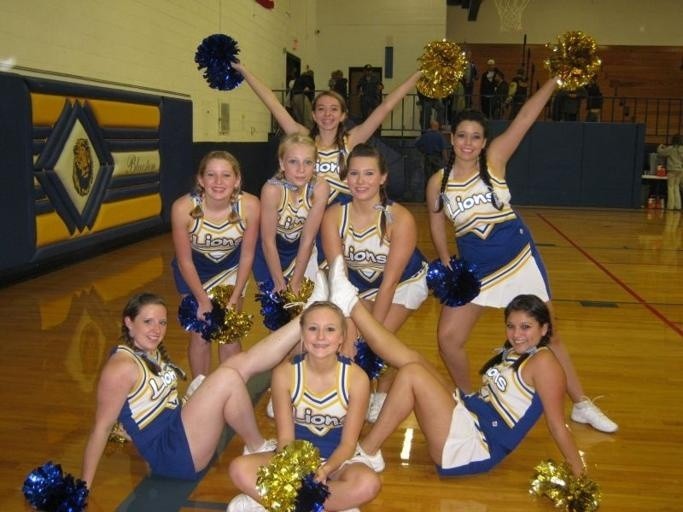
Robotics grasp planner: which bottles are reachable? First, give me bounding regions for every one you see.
[657,163,666,176]
[648,194,665,209]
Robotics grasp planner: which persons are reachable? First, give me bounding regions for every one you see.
[656,134,682,210]
[170,151,263,383]
[419,120,448,177]
[81,269,329,490]
[417,59,603,129]
[425,73,618,433]
[288,63,384,135]
[330,254,586,481]
[320,144,430,424]
[661,210,682,263]
[232,61,426,204]
[251,132,330,419]
[226,300,381,512]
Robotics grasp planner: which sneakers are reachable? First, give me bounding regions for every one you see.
[366,392,388,423]
[306,253,359,317]
[354,442,385,472]
[571,398,618,433]
[267,396,274,418]
[242,438,278,455]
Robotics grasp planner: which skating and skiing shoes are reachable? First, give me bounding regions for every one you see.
[226,493,273,512]
